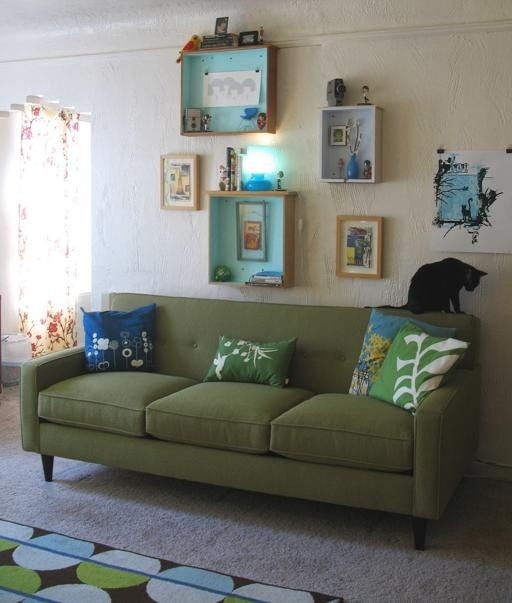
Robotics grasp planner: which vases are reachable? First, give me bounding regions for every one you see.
[347,155,359,179]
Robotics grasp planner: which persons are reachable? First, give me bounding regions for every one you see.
[217,21,226,33]
[245,226,258,249]
[206,77,257,105]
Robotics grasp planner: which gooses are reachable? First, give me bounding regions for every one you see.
[462,198,473,221]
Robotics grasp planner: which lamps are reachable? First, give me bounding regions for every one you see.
[241,145,276,191]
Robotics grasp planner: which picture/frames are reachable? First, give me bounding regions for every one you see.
[238,30,258,46]
[159,154,200,211]
[234,200,267,262]
[336,215,383,280]
[329,125,347,146]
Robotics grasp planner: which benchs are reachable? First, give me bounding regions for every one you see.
[19,290,483,549]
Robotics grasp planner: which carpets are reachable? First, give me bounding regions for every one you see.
[0,518,343,603]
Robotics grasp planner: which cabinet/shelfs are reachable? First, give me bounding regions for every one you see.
[204,190,298,289]
[318,105,384,184]
[179,43,277,137]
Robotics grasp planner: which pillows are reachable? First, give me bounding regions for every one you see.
[348,306,457,396]
[80,302,157,373]
[368,317,473,417]
[202,334,299,389]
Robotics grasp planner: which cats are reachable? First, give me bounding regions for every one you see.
[364,257,488,314]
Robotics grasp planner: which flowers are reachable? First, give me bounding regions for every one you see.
[344,117,362,154]
